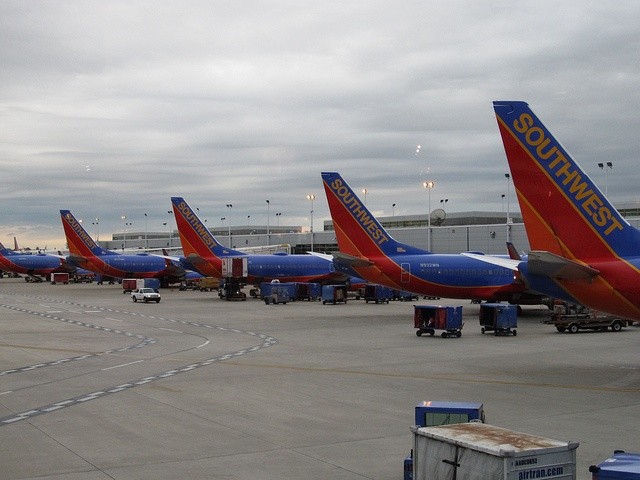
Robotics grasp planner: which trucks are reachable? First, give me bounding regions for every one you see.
[264,288,289,304]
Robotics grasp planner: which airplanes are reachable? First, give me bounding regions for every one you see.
[173,197,383,287]
[320,172,528,304]
[493,101,640,321]
[60,210,203,279]
[0,237,93,277]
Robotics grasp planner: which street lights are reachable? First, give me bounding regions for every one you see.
[392,203,396,215]
[501,194,505,212]
[307,194,315,251]
[226,204,232,249]
[220,218,226,227]
[163,223,167,232]
[361,188,368,207]
[504,172,510,255]
[266,199,270,246]
[144,214,147,249]
[422,182,434,252]
[126,223,132,239]
[441,198,449,214]
[204,220,207,225]
[276,212,281,227]
[92,222,99,242]
[196,208,200,217]
[96,217,100,242]
[598,162,613,198]
[168,210,173,247]
[248,215,250,235]
[121,216,126,249]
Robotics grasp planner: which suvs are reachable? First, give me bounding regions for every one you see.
[551,297,626,333]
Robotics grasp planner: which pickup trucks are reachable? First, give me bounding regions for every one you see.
[131,288,161,303]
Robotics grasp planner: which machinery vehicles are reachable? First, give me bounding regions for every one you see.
[145,279,160,293]
[295,283,319,301]
[122,279,145,294]
[51,273,69,285]
[413,304,465,339]
[322,285,348,305]
[589,449,640,480]
[480,304,517,336]
[404,401,579,480]
[364,284,390,304]
[199,277,220,292]
[260,282,296,302]
[389,288,419,302]
[218,276,246,301]
